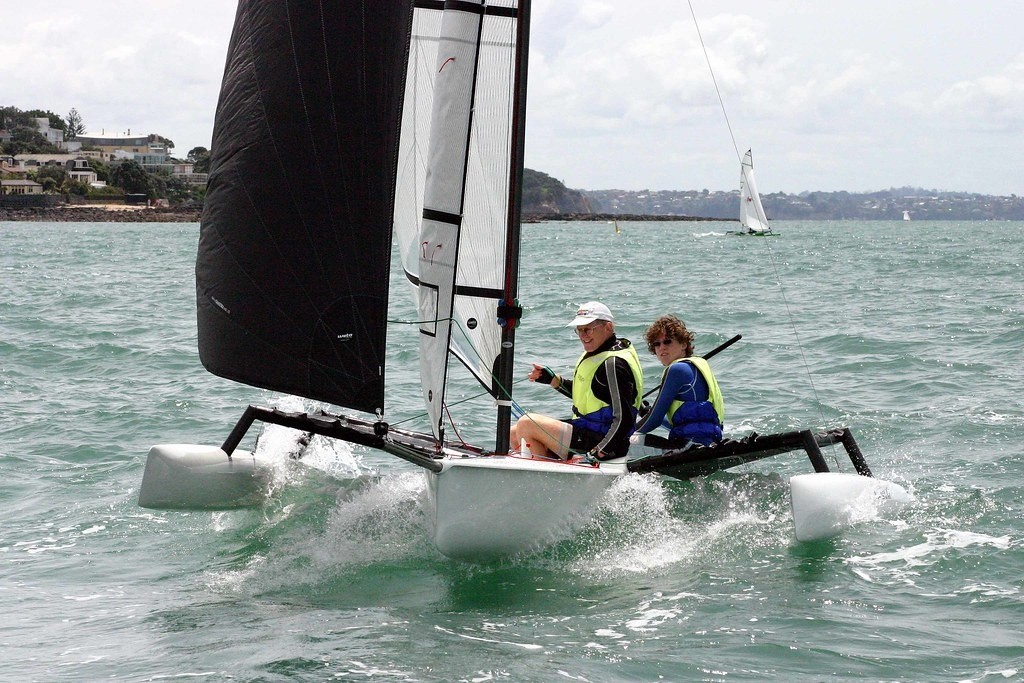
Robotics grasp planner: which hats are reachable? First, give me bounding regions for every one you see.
[566,301,614,328]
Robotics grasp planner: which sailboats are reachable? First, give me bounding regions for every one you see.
[722,145,783,240]
[130,1,922,563]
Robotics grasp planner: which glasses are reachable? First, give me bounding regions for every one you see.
[653,339,673,347]
[574,323,603,336]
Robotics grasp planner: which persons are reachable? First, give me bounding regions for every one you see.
[509,301,644,464]
[630,313,725,459]
